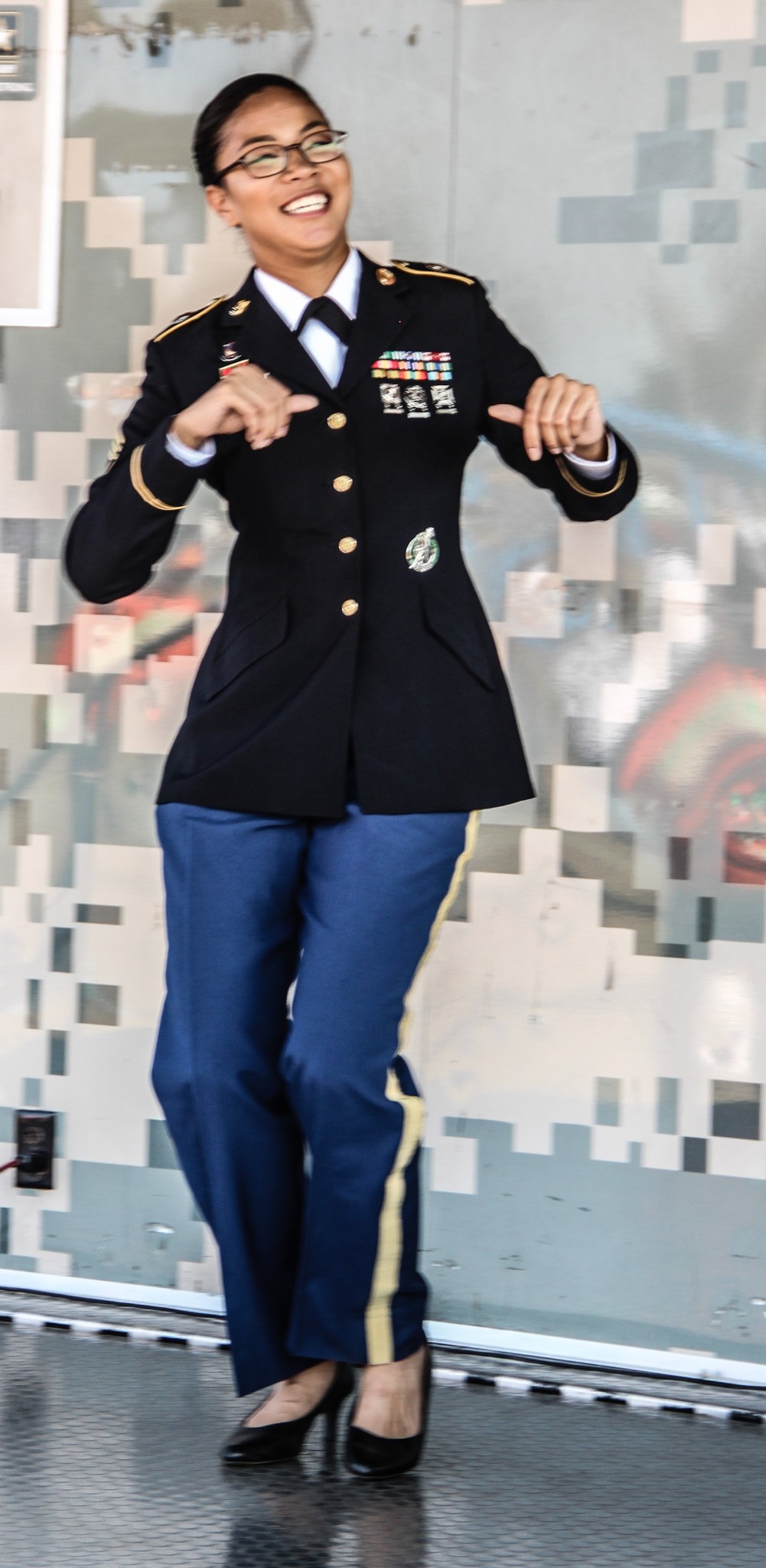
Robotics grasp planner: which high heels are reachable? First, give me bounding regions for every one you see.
[343,1342,431,1478]
[218,1361,355,1465]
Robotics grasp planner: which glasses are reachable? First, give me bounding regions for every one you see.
[201,129,348,185]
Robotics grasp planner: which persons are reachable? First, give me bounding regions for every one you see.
[66,74,640,1492]
